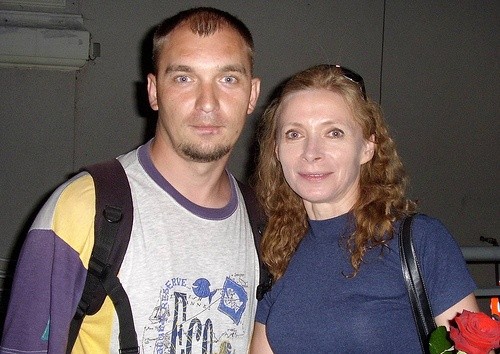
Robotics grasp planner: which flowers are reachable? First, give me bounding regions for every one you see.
[426,309,500,354]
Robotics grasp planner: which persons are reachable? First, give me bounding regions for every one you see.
[1,7,272,353]
[250,64,493,354]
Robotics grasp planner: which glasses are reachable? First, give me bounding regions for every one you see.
[321,64,368,104]
